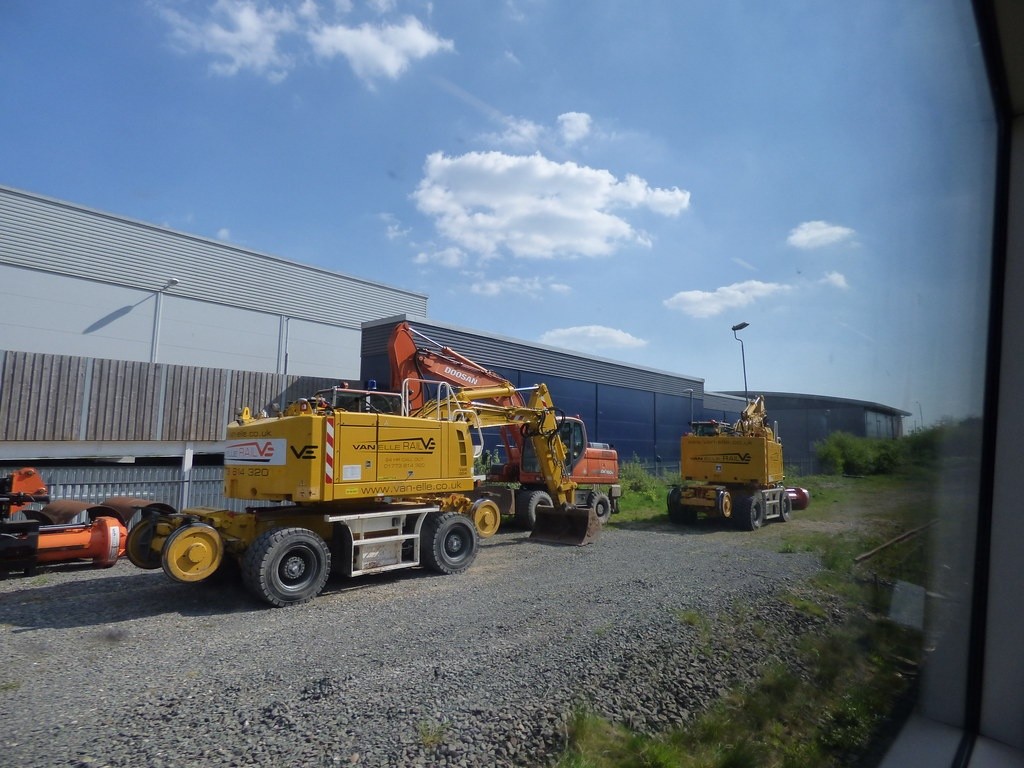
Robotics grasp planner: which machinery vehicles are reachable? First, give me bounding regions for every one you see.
[665,394,793,531]
[129,379,601,608]
[375,322,622,528]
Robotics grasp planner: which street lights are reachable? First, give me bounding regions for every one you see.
[732,321,751,410]
